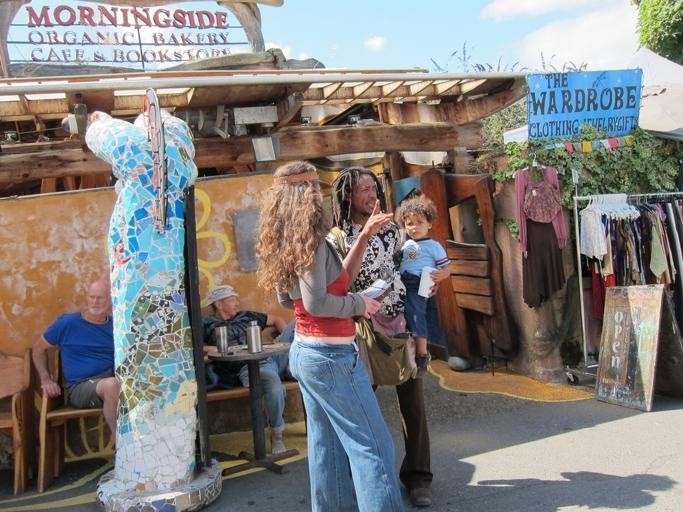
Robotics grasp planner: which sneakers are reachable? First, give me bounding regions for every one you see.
[414,351,432,380]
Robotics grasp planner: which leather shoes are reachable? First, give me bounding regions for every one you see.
[411,488,432,506]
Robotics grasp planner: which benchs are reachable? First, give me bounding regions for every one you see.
[36,341,300,493]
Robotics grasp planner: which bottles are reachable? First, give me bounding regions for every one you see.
[245,318,262,354]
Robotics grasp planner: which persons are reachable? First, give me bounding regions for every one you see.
[30,279,119,440]
[199,284,296,455]
[391,196,452,379]
[322,165,435,508]
[254,160,407,511]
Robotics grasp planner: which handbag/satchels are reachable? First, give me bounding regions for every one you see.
[356,313,419,386]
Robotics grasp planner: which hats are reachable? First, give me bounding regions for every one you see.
[200,285,238,308]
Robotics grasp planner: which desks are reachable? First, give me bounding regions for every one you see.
[205,345,304,477]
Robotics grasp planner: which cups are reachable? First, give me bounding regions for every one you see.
[417,266,437,299]
[214,326,229,354]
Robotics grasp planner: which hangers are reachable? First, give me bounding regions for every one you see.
[575,189,682,221]
[506,152,564,179]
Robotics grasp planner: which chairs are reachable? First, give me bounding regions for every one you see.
[0,348,31,496]
